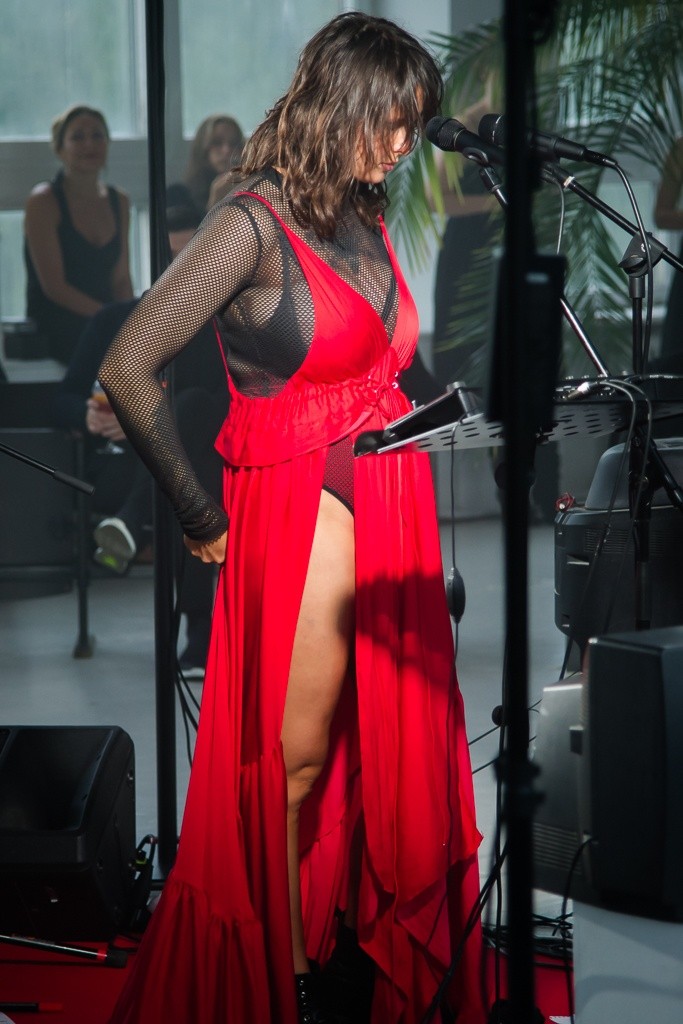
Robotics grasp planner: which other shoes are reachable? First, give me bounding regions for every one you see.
[93,517,137,574]
[293,974,316,1024]
[178,652,206,677]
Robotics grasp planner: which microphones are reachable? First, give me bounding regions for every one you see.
[479,114,617,167]
[426,115,556,183]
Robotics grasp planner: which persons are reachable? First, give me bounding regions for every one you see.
[23,107,248,678]
[98,12,489,1024]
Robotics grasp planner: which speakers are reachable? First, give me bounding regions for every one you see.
[0,724,137,942]
[584,625,683,926]
